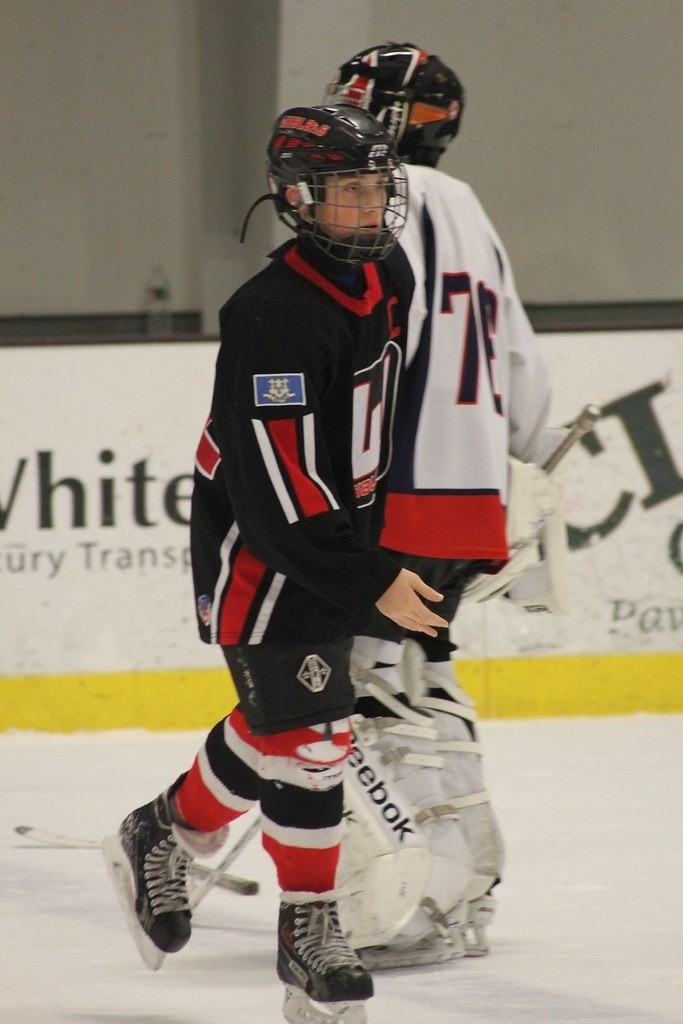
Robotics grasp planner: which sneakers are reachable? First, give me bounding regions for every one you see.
[347,897,493,970]
[103,770,231,971]
[277,886,373,1024]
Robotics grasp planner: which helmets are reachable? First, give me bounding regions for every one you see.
[323,42,465,168]
[268,103,410,264]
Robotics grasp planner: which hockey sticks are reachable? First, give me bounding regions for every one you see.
[10,404,599,970]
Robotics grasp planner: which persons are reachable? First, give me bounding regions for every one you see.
[103,100,447,1024]
[325,44,570,974]
